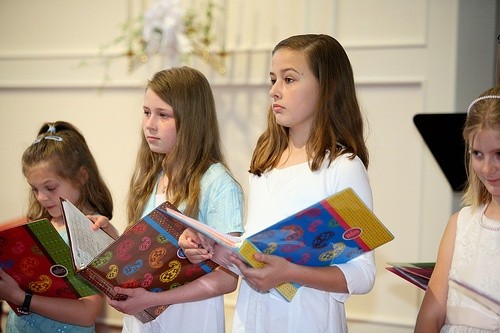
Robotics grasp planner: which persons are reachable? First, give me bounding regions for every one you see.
[86,65,244,333]
[412,87,500,333]
[177,33,376,333]
[0,121,104,333]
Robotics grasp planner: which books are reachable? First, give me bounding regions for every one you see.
[448,279,500,316]
[58,196,220,324]
[384,260,437,292]
[157,186,395,303]
[0,218,102,300]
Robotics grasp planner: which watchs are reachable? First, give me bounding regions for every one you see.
[16,290,33,314]
